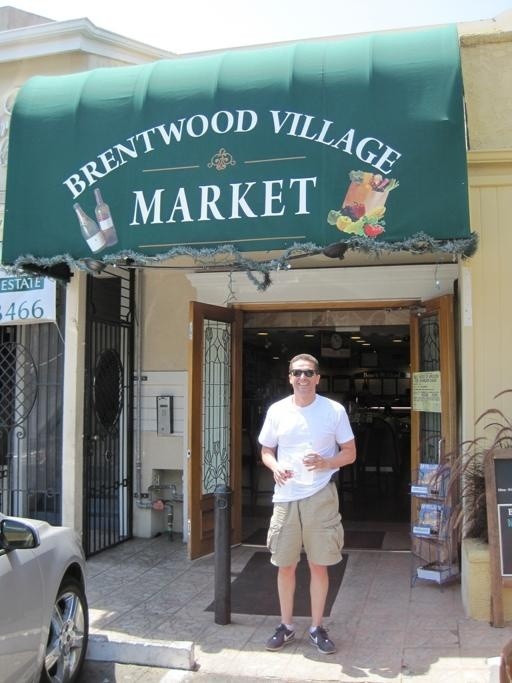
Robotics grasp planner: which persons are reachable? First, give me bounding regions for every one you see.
[257,353,358,655]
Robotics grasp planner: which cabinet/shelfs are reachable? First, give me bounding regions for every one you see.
[408,433,460,594]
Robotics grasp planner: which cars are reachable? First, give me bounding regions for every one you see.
[0,508,89,683]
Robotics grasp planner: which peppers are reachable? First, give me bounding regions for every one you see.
[327,202,383,237]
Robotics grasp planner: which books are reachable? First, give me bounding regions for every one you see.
[410,462,459,583]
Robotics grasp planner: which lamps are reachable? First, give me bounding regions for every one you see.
[85,258,130,281]
[322,242,347,260]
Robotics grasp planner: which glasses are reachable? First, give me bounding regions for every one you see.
[288,369,314,378]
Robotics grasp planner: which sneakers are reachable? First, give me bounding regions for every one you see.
[264,624,296,651]
[308,625,337,654]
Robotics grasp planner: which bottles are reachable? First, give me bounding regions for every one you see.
[302,442,313,481]
[72,202,106,255]
[93,188,119,248]
